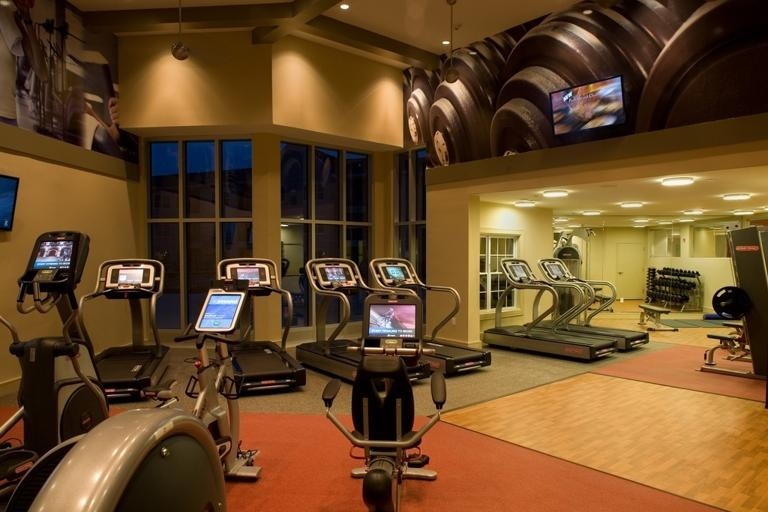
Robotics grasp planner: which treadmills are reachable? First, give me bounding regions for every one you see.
[296,257,492,385]
[481,257,649,361]
[215,257,307,393]
[77,258,172,403]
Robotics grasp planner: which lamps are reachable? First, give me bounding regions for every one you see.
[445,0,460,84]
[171,0,189,60]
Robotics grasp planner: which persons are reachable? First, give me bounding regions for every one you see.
[0,0,121,153]
[380,305,400,322]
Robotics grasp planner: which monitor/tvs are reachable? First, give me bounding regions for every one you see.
[376,263,415,284]
[105,263,155,291]
[363,299,424,341]
[547,74,636,148]
[225,263,271,285]
[195,288,245,332]
[542,262,568,279]
[315,263,358,287]
[0,174,20,231]
[30,238,76,274]
[506,262,533,282]
